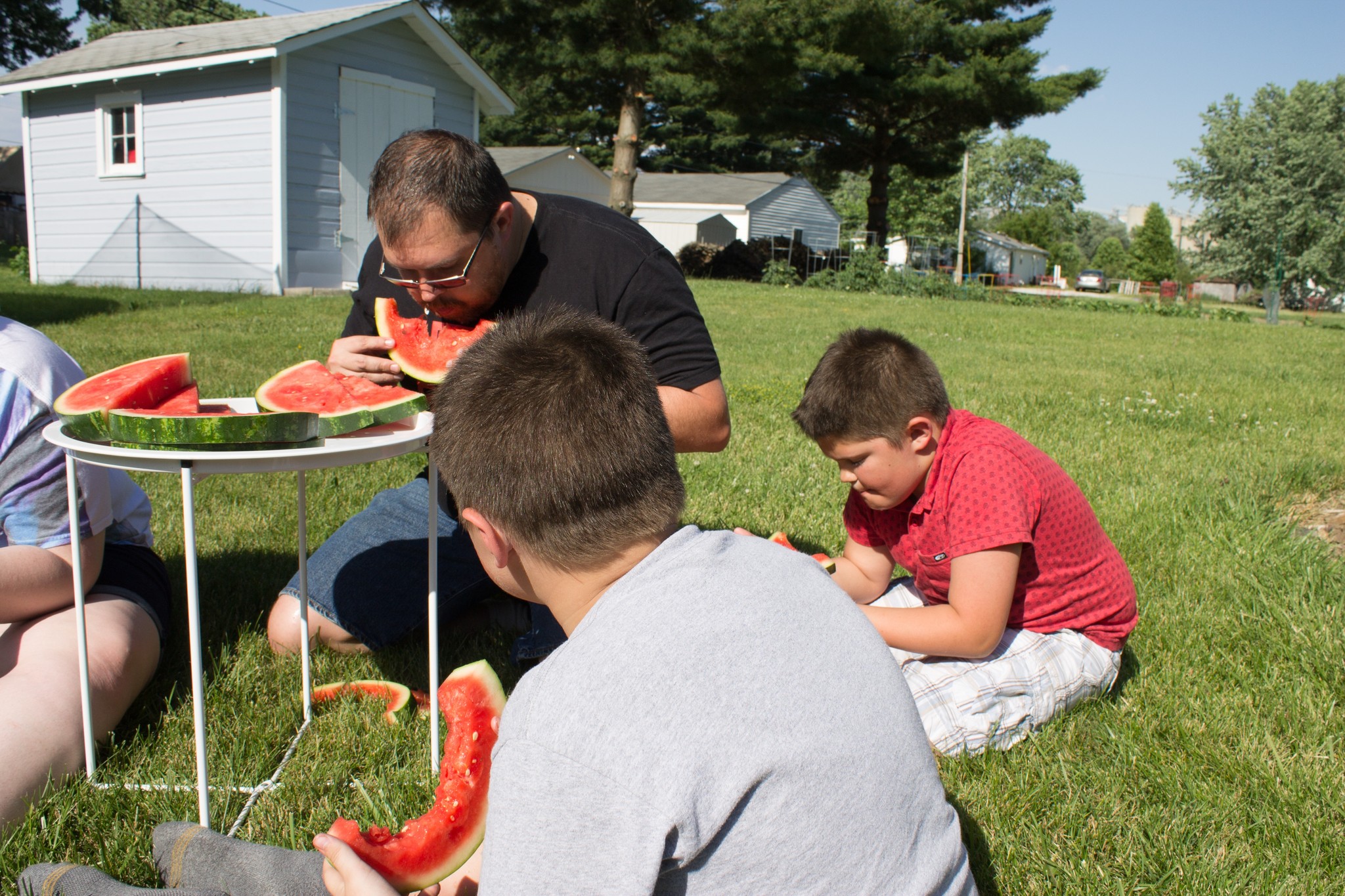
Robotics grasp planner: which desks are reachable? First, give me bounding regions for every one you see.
[40,394,440,839]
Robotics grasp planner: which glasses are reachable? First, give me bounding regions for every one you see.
[379,218,492,289]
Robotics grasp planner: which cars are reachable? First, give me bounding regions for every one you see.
[1076,269,1111,294]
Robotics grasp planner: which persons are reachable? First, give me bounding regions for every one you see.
[18,308,981,896]
[733,326,1141,757]
[268,132,731,694]
[0,315,169,838]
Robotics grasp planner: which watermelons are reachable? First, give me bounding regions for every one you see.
[52,352,325,451]
[331,370,430,430]
[374,297,500,385]
[328,657,508,893]
[767,531,836,575]
[254,358,374,438]
